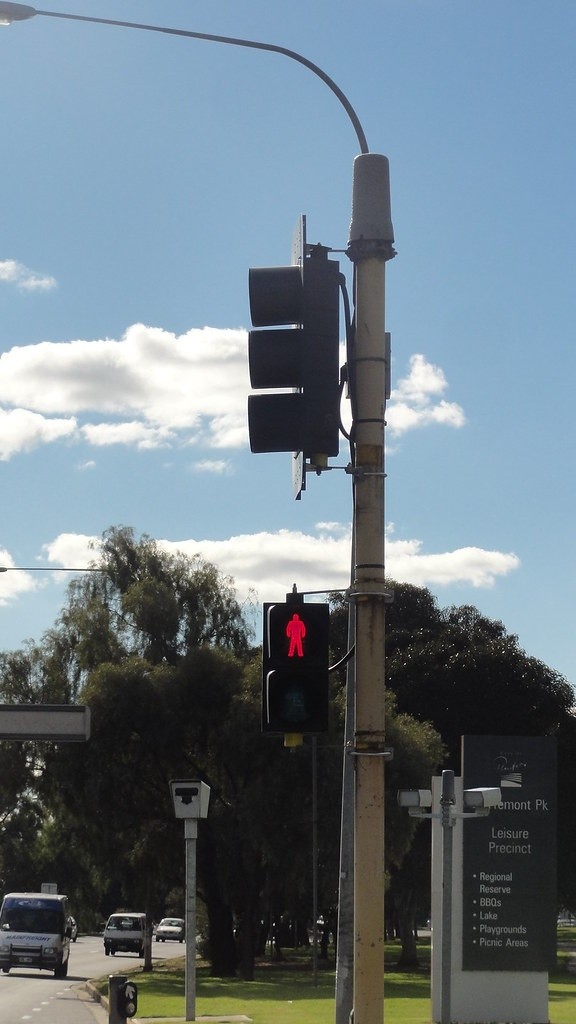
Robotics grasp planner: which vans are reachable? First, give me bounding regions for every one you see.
[103,911,146,958]
[0,892,69,978]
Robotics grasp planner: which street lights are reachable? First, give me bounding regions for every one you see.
[0,3,383,1024]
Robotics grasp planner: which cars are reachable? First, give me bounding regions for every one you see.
[70,916,78,943]
[156,918,184,943]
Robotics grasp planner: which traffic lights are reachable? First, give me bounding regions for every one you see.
[250,214,337,498]
[262,603,334,736]
